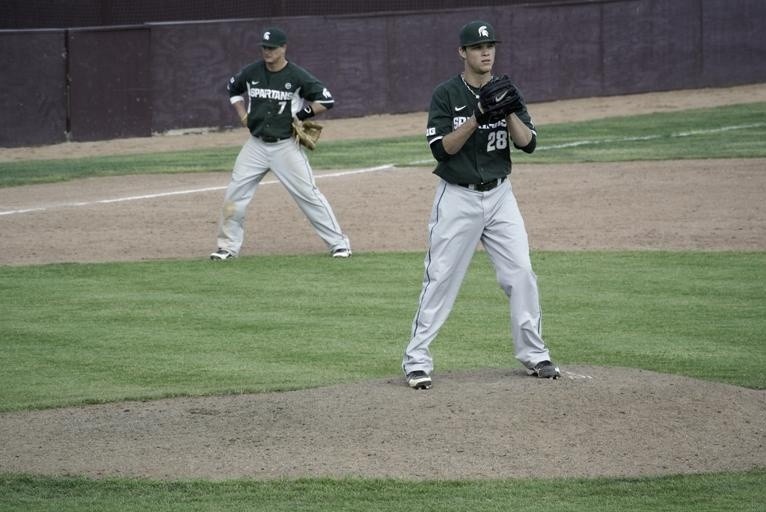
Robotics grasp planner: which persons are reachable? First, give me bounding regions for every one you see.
[399,21,567,392]
[207,28,354,261]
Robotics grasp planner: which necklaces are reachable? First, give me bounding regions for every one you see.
[458,72,495,98]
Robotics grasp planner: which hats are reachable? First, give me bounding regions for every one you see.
[254,29,287,48]
[459,20,502,46]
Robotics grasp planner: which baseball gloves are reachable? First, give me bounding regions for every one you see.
[475,74,521,125]
[294,119,323,150]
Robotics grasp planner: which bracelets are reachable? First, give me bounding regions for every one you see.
[241,112,248,123]
[296,105,316,122]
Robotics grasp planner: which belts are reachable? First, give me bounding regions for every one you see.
[253,134,289,142]
[457,176,505,191]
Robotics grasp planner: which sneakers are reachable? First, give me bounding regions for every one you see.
[209,250,232,260]
[404,370,431,390]
[526,360,560,378]
[332,248,351,258]
